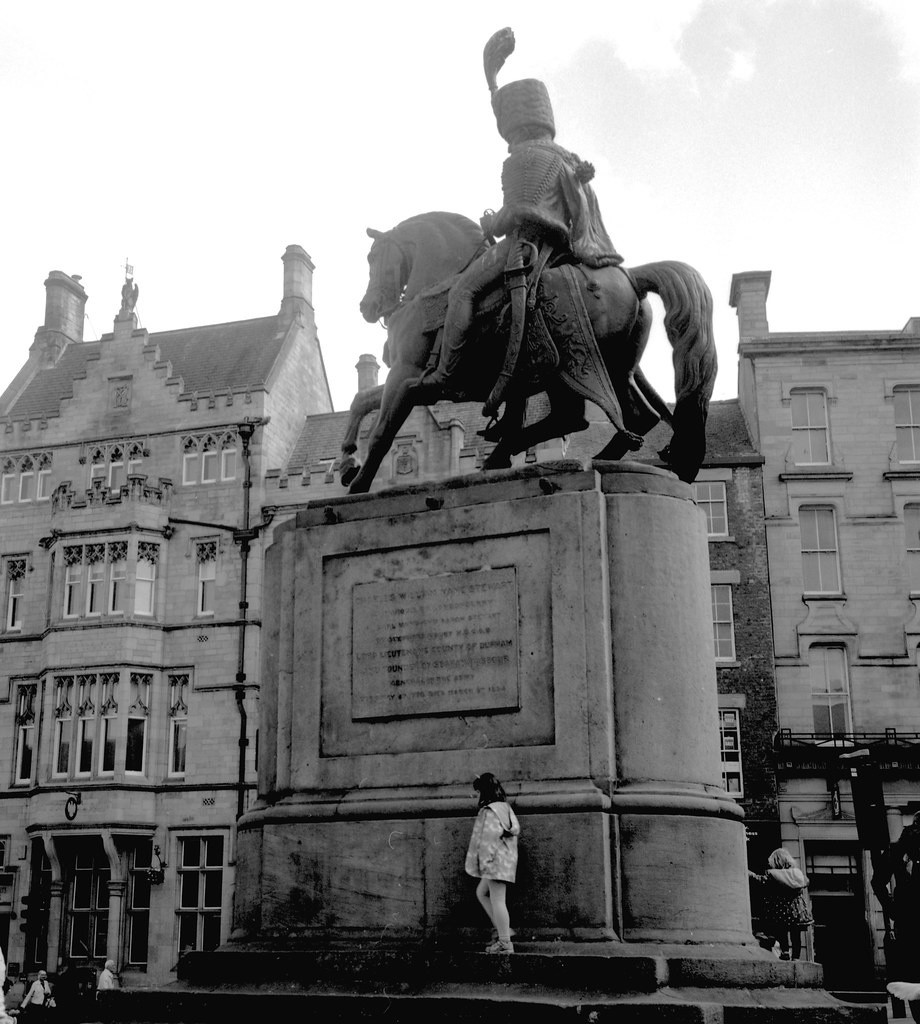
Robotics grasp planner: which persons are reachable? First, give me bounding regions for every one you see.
[763,847,815,961]
[406,125,625,389]
[19,970,51,1024]
[463,774,521,956]
[95,959,116,1000]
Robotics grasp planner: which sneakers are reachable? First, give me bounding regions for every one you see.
[484,937,515,955]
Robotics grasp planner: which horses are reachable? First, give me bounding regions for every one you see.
[337,209,719,486]
